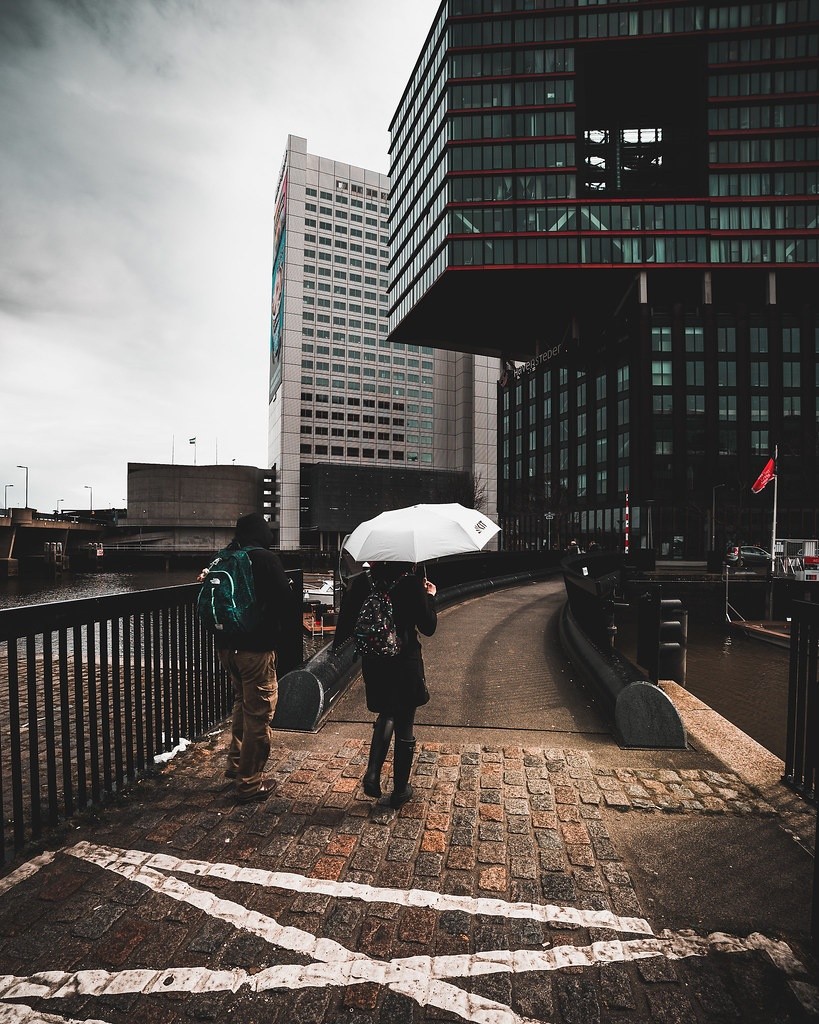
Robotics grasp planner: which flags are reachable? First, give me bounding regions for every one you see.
[751,458,775,493]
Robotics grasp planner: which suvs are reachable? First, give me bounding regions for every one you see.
[725,546,776,567]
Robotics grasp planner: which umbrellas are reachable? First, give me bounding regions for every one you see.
[344,502,502,577]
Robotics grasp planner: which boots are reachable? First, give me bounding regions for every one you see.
[389,738,418,805]
[363,736,390,798]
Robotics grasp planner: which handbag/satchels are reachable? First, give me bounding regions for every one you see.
[577,545,581,554]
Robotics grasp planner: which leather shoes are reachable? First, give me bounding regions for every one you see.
[239,778,278,804]
[225,770,238,779]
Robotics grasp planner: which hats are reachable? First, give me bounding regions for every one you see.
[571,541,577,544]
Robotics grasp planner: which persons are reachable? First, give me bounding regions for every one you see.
[567,541,580,554]
[727,543,738,574]
[340,562,437,807]
[590,541,602,551]
[217,514,297,802]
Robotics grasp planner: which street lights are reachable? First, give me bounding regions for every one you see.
[5,485,13,517]
[712,484,724,550]
[17,466,27,508]
[86,486,92,510]
[544,512,555,550]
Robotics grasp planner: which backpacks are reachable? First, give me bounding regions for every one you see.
[193,543,266,638]
[350,570,413,663]
